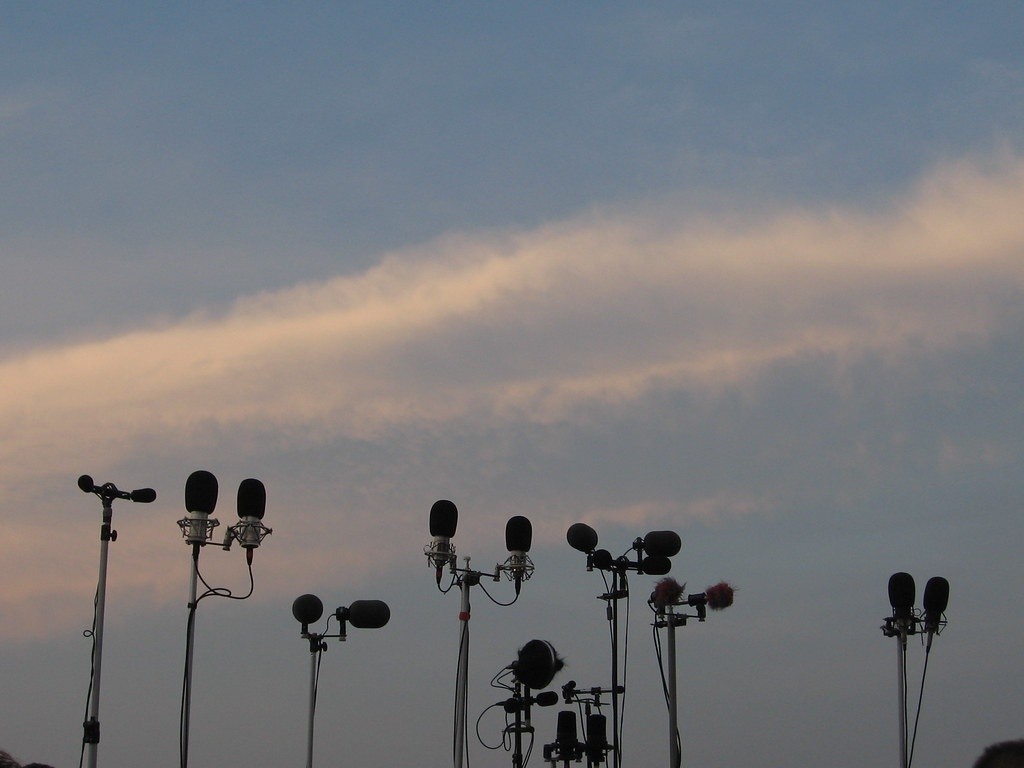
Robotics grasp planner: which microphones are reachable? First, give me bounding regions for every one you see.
[131,488,156,503]
[923,577,950,651]
[567,523,733,609]
[292,594,325,625]
[336,600,390,628]
[237,479,266,563]
[498,640,607,768]
[78,475,94,492]
[430,500,458,584]
[506,516,532,594]
[185,470,219,560]
[889,572,916,648]
